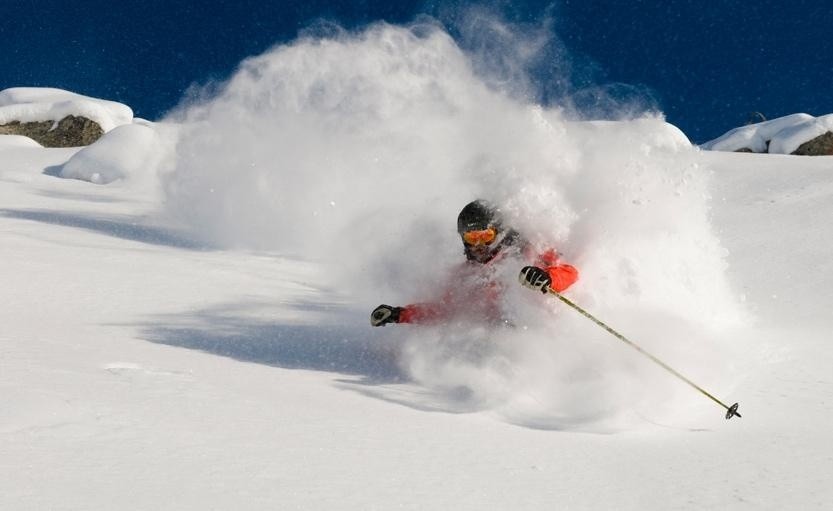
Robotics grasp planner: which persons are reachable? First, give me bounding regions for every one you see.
[368,197,580,333]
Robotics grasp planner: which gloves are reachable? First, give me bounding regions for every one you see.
[370,304,400,327]
[518,266,552,294]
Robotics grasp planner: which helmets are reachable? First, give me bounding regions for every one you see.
[456,199,502,236]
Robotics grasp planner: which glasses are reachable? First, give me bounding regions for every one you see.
[459,227,500,245]
